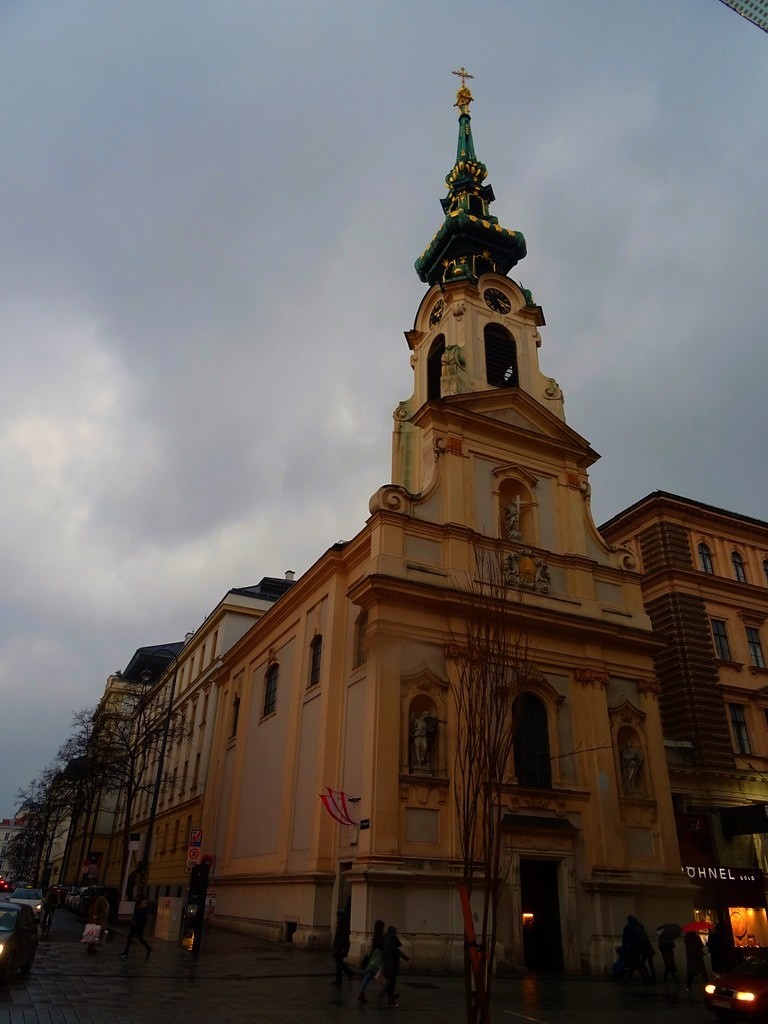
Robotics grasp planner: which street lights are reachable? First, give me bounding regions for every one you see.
[136,649,179,907]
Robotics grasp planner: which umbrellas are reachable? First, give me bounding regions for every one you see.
[655,923,683,940]
[681,921,714,933]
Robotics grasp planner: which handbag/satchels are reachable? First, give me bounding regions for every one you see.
[80,918,102,945]
[369,949,384,973]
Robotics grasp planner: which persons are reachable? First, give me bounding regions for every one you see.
[504,498,519,533]
[658,936,683,986]
[86,888,110,954]
[685,931,710,992]
[412,711,430,765]
[706,923,730,976]
[330,909,353,987]
[613,914,656,986]
[621,740,644,791]
[357,919,413,1007]
[117,890,153,960]
[40,886,57,928]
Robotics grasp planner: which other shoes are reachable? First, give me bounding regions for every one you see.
[331,980,342,987]
[119,953,128,957]
[384,999,400,1008]
[347,970,354,982]
[358,993,368,1004]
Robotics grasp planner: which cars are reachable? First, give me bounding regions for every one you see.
[0,902,39,987]
[6,885,46,924]
[703,949,768,1024]
[54,883,120,922]
